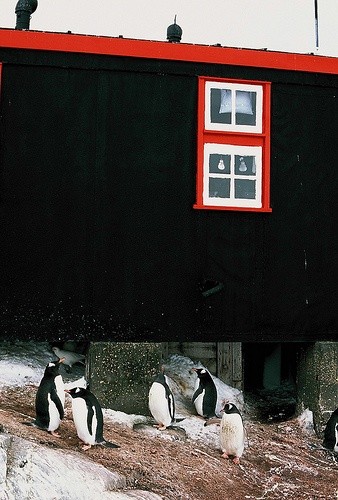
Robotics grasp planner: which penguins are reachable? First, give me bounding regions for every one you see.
[322,408,338,453]
[64,387,121,451]
[204,403,249,464]
[21,357,65,438]
[148,367,187,431]
[190,368,218,421]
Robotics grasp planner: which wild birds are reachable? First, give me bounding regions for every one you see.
[50,346,86,374]
